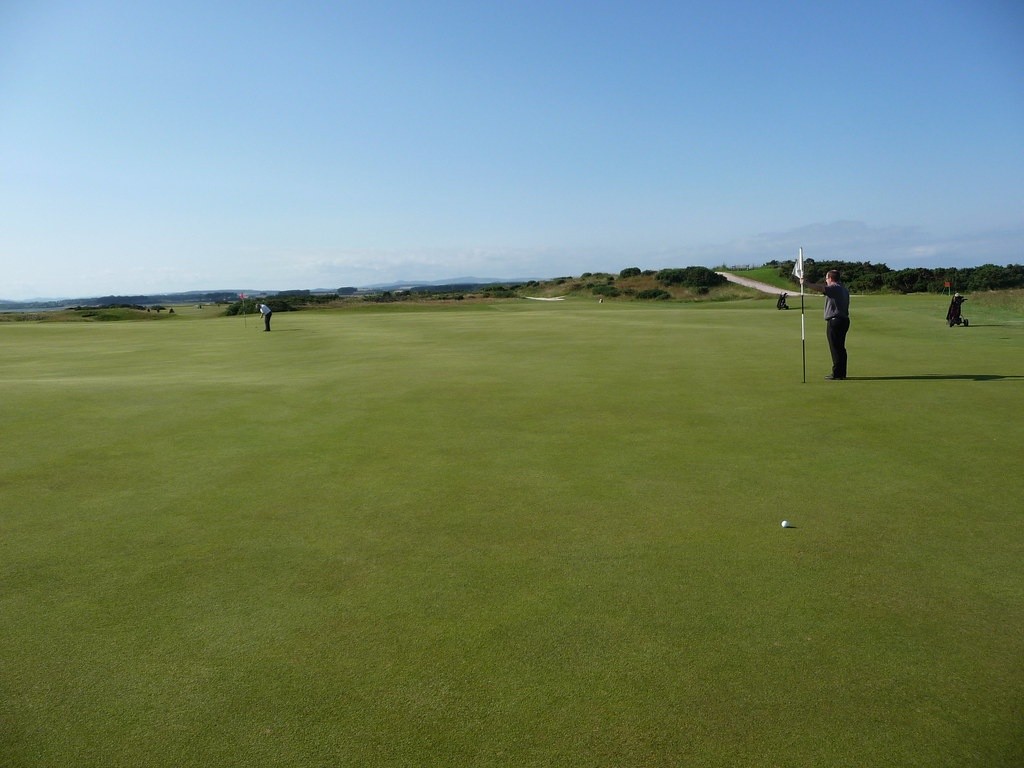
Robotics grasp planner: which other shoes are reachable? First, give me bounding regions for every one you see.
[825,374,846,380]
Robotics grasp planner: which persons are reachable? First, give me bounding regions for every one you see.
[946,293,964,321]
[800,270,850,380]
[256,304,271,331]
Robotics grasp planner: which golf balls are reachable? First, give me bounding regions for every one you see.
[781,521,789,528]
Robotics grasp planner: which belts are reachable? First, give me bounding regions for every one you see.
[829,316,843,320]
